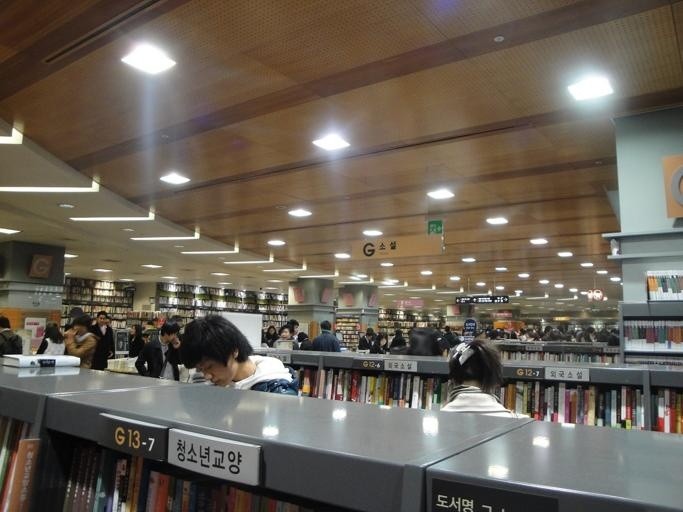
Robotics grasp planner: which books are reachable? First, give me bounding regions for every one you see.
[290,364,317,397]
[335,316,360,352]
[650,386,683,435]
[625,355,682,365]
[378,308,446,346]
[319,367,451,411]
[125,310,177,345]
[156,282,289,333]
[59,276,135,338]
[494,379,644,431]
[0,416,34,512]
[646,269,683,301]
[106,356,148,374]
[0,353,80,367]
[38,428,326,512]
[498,350,621,365]
[622,319,683,353]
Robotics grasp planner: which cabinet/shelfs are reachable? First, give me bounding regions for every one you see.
[600,227,683,364]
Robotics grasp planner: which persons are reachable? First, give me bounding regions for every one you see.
[313,319,341,352]
[171,315,185,334]
[367,327,377,337]
[91,311,115,359]
[0,316,22,357]
[105,316,112,327]
[65,306,83,330]
[440,339,522,418]
[180,315,300,396]
[63,314,97,369]
[273,324,299,350]
[370,334,390,355]
[285,318,300,342]
[297,332,312,351]
[390,330,406,347]
[135,319,181,381]
[264,326,279,348]
[36,322,66,355]
[442,323,619,349]
[359,332,373,350]
[129,324,145,359]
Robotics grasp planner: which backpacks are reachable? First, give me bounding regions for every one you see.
[91,335,113,370]
[0,332,21,354]
